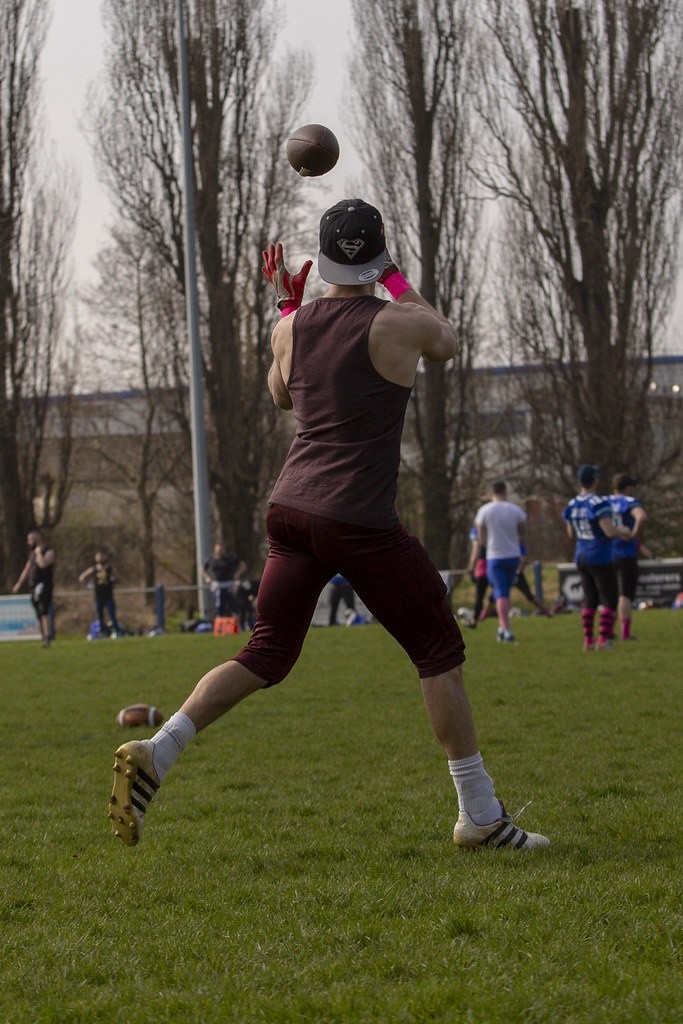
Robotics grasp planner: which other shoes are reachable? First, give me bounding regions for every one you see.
[496,630,515,642]
[462,620,477,628]
[478,609,487,621]
[540,607,552,617]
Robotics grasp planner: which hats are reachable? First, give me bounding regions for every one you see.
[318,198,387,285]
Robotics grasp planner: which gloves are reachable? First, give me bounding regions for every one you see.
[261,242,313,318]
[377,246,413,301]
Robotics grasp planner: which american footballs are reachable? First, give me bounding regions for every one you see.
[286,123,341,178]
[115,702,165,730]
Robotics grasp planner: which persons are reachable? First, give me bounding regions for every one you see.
[473,481,529,644]
[460,526,489,629]
[600,471,647,640]
[236,579,262,631]
[479,542,553,624]
[108,198,552,853]
[79,550,124,638]
[327,574,356,627]
[202,543,247,618]
[13,531,54,649]
[561,466,630,651]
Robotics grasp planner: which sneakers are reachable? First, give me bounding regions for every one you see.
[453,799,551,849]
[107,738,161,846]
